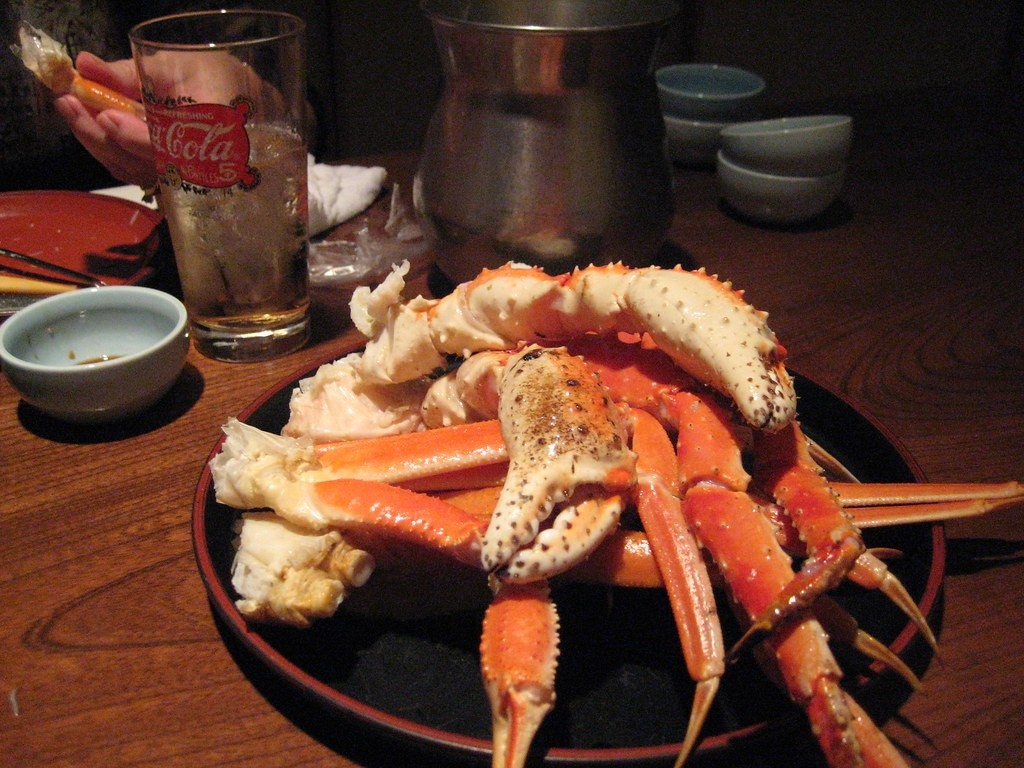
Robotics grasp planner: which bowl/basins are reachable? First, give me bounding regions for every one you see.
[720,114,854,177]
[654,63,765,121]
[662,117,745,169]
[0,286,191,423]
[717,148,845,222]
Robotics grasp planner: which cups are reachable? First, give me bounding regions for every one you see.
[128,9,313,361]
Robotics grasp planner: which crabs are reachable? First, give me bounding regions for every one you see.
[206,252,1024,768]
[5,17,148,124]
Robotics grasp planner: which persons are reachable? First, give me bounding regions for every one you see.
[0,0,338,193]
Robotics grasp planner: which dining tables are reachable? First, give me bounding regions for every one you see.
[0,69,1023,768]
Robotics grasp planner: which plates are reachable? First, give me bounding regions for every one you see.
[191,337,951,768]
[0,190,176,319]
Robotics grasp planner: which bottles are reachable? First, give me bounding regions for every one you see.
[412,3,670,307]
